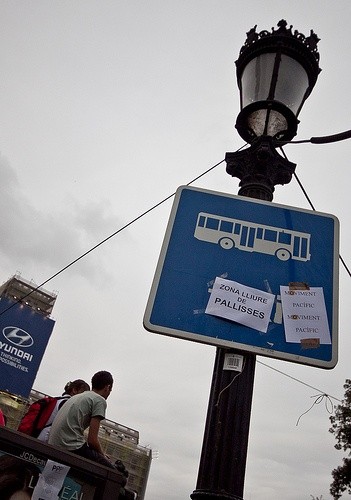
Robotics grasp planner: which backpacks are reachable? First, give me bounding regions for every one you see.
[18,396,71,439]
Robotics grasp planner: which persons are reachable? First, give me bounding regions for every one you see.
[33,379,91,445]
[47,369,138,500]
[0,452,43,500]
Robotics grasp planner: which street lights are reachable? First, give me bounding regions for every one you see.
[191,19,323,500]
